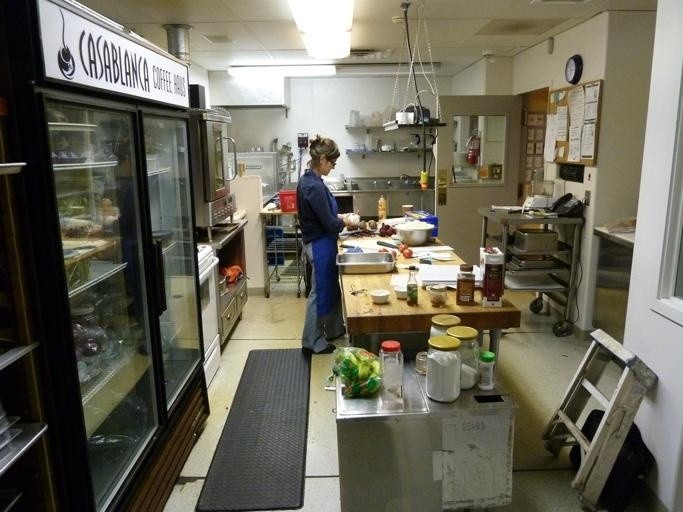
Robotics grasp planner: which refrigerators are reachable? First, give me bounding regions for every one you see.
[17,0,209,510]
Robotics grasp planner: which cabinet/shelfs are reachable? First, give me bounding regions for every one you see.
[343,124,440,154]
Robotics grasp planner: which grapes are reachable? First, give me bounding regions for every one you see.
[380,223,395,236]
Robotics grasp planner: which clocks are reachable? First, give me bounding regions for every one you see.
[564,54,582,85]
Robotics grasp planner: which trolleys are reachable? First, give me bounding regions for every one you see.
[476,209,584,336]
[261,200,308,298]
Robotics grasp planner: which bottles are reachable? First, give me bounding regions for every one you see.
[406,264,474,306]
[426,314,495,402]
[378,340,404,410]
[377,195,386,220]
[468,129,480,165]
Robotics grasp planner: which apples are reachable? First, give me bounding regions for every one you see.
[403,249,412,257]
[399,240,409,252]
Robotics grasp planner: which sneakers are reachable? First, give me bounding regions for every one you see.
[302,344,336,354]
[326,325,346,341]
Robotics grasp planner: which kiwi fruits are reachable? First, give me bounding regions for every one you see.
[370,220,376,229]
[368,219,374,227]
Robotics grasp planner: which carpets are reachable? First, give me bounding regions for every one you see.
[195,346,312,510]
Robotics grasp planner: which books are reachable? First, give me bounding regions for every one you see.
[417,264,481,290]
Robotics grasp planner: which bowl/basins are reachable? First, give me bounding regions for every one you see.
[370,289,389,305]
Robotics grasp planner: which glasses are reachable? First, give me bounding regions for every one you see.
[326,155,336,167]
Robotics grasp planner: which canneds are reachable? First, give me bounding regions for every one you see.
[430,286,449,308]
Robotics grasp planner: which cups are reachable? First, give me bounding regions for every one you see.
[402,204,413,216]
[426,285,448,307]
[415,351,427,374]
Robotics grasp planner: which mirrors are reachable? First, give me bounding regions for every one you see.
[450,112,508,186]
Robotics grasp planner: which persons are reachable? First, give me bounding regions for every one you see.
[296,134,361,354]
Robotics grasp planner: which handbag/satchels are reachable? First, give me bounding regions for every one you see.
[570,410,655,512]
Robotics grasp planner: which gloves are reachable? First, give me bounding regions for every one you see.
[337,213,360,226]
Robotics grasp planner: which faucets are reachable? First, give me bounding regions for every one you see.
[400,173,417,185]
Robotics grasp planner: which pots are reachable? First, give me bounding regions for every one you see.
[392,223,437,246]
